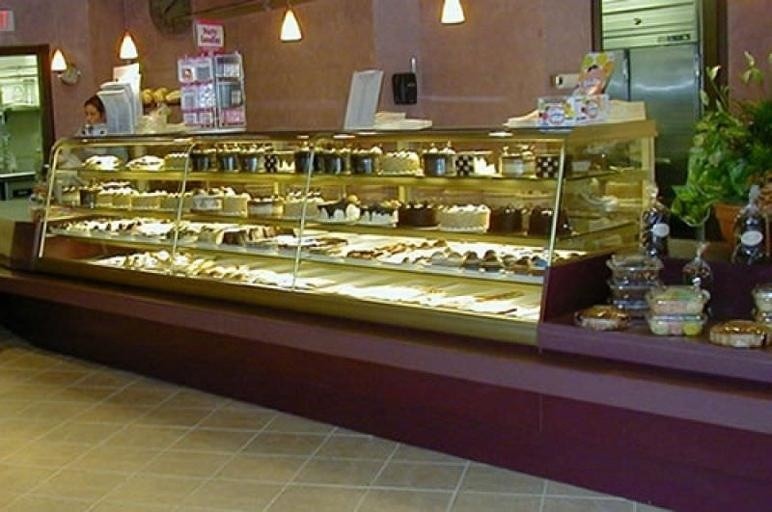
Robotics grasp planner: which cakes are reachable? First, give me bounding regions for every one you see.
[121,248,250,285]
[191,143,561,181]
[127,154,163,172]
[64,183,568,239]
[81,153,124,173]
[163,152,191,171]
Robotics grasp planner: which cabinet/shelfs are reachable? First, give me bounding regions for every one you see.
[668,52,772,260]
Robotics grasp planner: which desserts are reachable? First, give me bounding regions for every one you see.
[61,216,577,276]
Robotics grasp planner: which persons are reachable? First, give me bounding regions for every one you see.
[76,94,108,138]
[576,64,601,88]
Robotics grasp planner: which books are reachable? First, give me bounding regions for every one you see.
[569,48,625,97]
[100,81,137,127]
[96,89,135,134]
